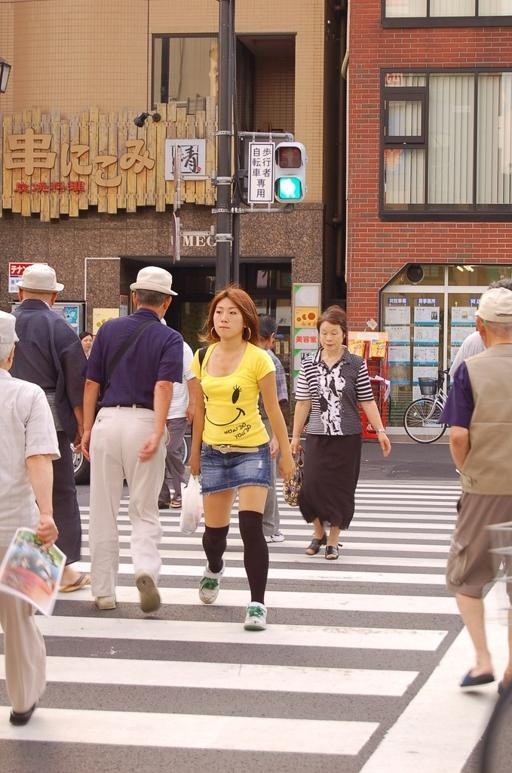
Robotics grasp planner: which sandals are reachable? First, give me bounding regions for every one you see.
[304,531,326,554]
[326,542,339,559]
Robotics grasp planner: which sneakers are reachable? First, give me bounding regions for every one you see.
[135,572,161,613]
[157,501,169,510]
[264,532,285,542]
[244,601,267,631]
[170,495,184,509]
[198,561,225,604]
[93,596,118,609]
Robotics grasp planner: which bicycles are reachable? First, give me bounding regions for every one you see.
[403,367,449,444]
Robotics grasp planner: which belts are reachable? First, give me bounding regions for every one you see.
[94,399,151,411]
[210,445,260,454]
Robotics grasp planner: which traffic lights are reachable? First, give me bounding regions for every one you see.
[274,142,307,203]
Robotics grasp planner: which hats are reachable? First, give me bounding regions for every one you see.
[129,266,178,295]
[18,262,65,293]
[0,311,20,344]
[475,287,512,326]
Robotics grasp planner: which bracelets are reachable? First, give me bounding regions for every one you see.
[372,429,387,433]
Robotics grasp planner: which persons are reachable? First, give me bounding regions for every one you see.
[437,288,512,694]
[0,312,60,727]
[81,266,181,612]
[4,263,87,594]
[81,334,92,354]
[188,289,295,630]
[260,318,290,543]
[449,322,486,382]
[289,308,390,559]
[157,319,200,506]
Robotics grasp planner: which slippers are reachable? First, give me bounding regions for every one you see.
[456,665,499,692]
[58,571,90,592]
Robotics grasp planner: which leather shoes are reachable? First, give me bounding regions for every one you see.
[9,703,35,725]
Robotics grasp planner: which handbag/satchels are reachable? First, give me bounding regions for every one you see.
[283,446,305,507]
